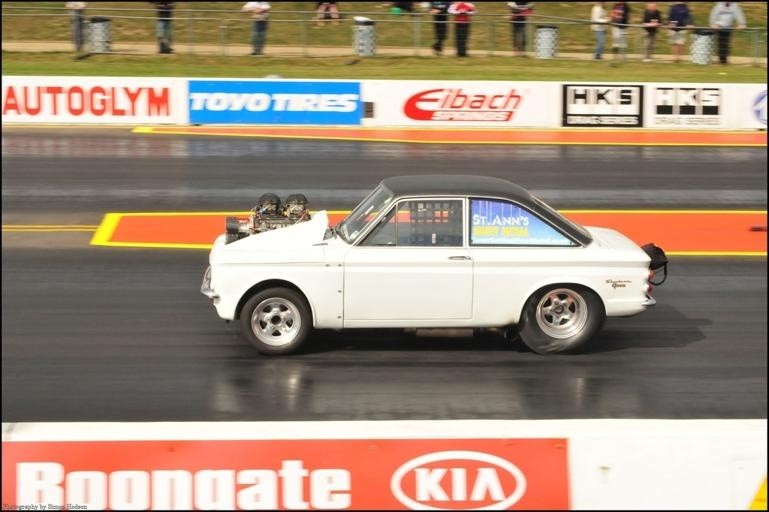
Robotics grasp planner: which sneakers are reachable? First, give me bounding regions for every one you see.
[249,50,266,58]
[157,43,176,57]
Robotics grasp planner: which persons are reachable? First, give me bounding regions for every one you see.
[447,1,475,57]
[430,0,450,52]
[156,1,175,53]
[508,0,535,52]
[591,2,747,64]
[242,1,271,55]
[316,0,340,27]
[66,0,86,51]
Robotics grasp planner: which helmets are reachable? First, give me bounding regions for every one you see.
[285,194,309,205]
[258,193,281,206]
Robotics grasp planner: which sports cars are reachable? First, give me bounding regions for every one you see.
[198,173,673,364]
